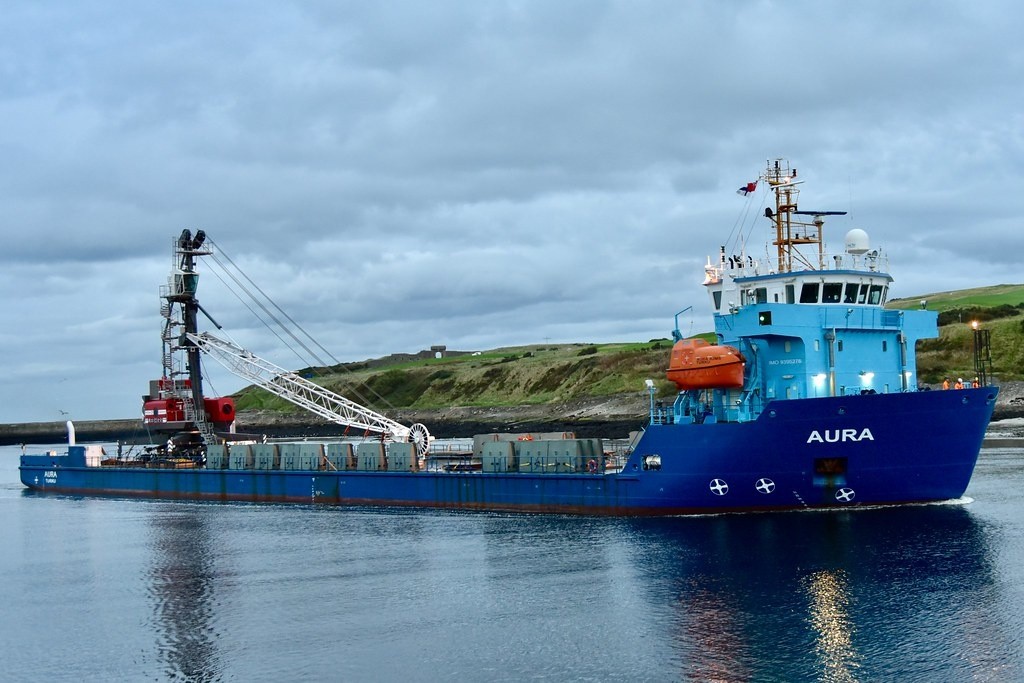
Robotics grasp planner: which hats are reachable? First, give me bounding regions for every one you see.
[958,378,962,383]
[974,377,978,381]
[944,376,950,380]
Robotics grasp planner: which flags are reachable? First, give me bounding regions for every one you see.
[747,179,758,191]
[736,186,748,196]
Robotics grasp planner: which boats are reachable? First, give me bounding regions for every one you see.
[17,158,1000,512]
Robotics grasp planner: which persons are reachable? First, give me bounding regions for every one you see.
[972,377,979,388]
[954,377,964,389]
[943,377,950,390]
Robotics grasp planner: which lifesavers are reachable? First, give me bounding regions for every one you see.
[587,459,597,474]
